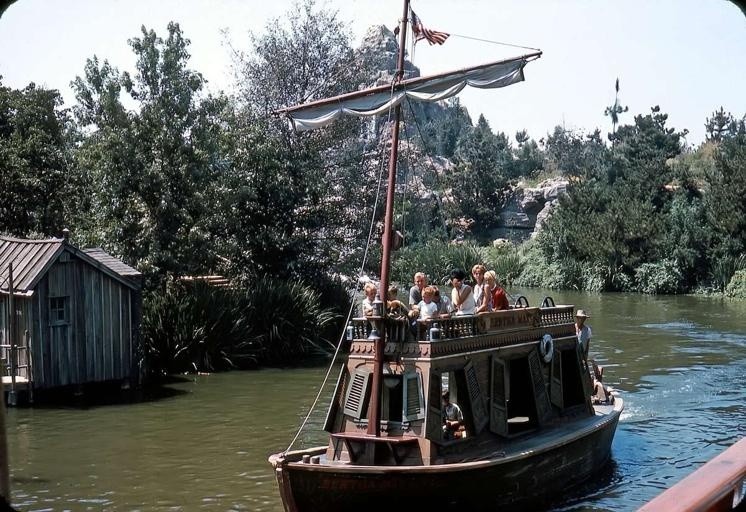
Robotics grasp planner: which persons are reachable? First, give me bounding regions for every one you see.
[362,263,510,340]
[573,309,592,363]
[440,387,469,442]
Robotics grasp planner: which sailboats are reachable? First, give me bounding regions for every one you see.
[266,0,626,511]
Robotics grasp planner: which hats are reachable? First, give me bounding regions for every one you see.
[574,310,590,318]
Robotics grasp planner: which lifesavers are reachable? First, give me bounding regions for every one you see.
[539,334,554,363]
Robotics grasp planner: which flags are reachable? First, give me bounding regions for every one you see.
[411,11,451,47]
